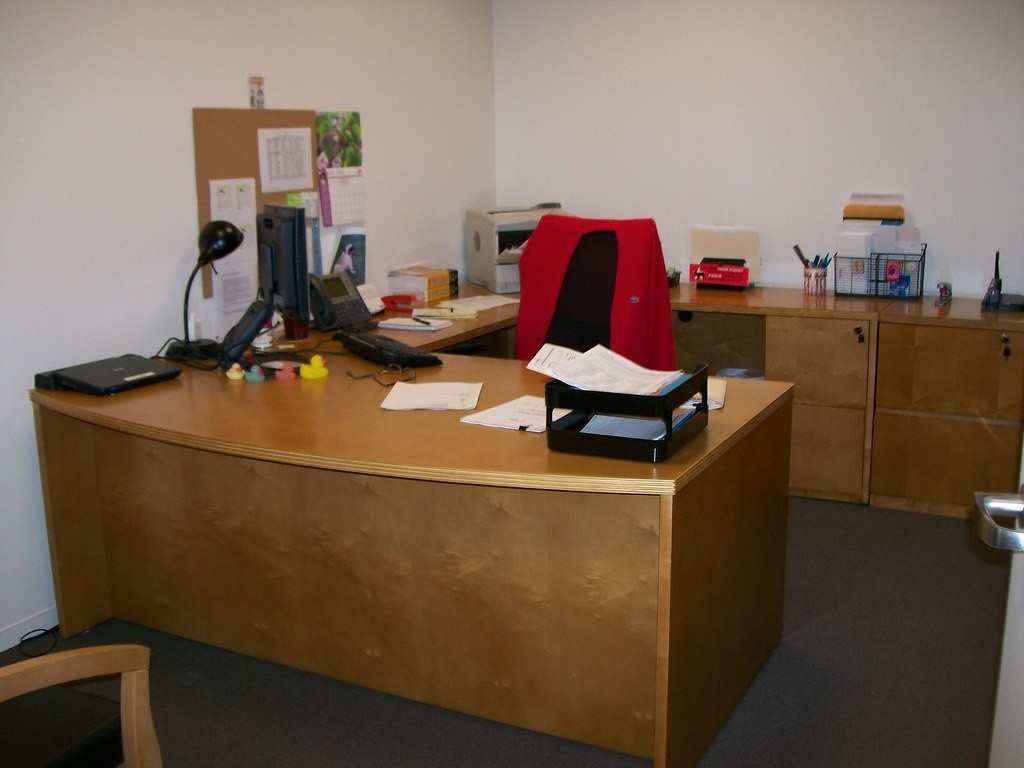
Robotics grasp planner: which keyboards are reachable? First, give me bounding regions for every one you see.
[344,335,443,371]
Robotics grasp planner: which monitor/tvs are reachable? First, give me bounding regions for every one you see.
[218,203,310,375]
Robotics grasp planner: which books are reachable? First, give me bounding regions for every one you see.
[388,265,458,303]
[678,379,728,410]
[378,308,478,331]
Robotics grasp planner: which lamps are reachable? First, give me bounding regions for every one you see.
[166,220,243,360]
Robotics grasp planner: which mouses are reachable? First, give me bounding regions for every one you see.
[332,330,350,340]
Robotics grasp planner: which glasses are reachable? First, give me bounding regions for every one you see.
[346,363,416,387]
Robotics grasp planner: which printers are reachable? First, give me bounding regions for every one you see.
[466,202,568,294]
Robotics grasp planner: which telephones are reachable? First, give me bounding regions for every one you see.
[308,271,371,332]
[981,251,1024,312]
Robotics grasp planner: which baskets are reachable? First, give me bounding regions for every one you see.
[834,243,927,299]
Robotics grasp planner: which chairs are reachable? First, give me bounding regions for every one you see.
[0,643,162,768]
[516,213,670,375]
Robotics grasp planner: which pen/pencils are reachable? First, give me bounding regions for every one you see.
[805,252,832,268]
[412,317,430,325]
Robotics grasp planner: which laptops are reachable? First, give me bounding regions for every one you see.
[52,353,182,396]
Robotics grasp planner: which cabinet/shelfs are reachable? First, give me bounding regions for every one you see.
[868,296,1023,516]
[669,282,897,507]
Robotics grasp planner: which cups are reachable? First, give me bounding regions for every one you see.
[803,268,827,296]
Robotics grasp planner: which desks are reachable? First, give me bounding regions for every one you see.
[29,283,796,768]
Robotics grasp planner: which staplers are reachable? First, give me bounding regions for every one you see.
[380,294,416,312]
